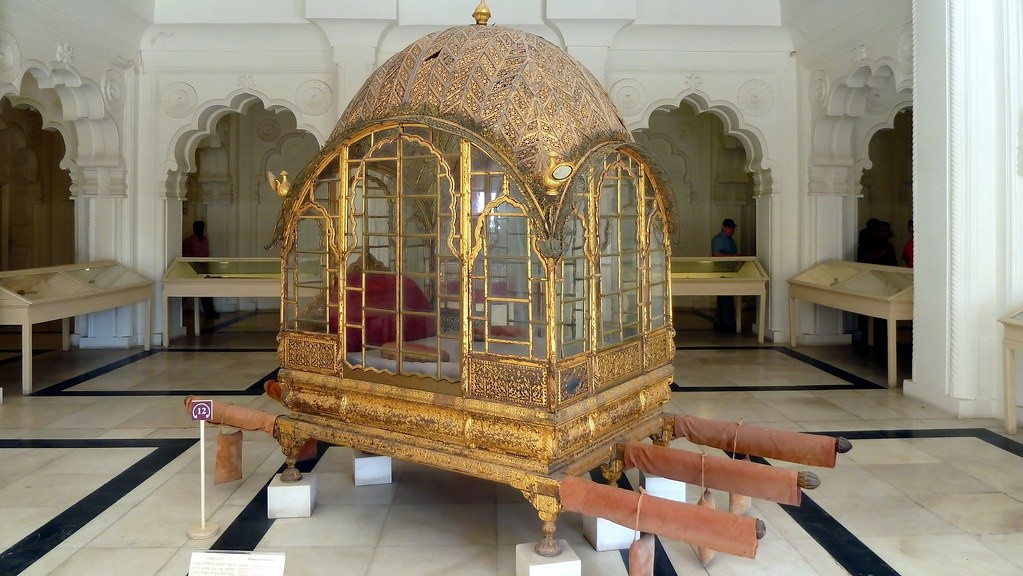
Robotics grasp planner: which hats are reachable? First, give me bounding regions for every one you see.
[723,219,737,228]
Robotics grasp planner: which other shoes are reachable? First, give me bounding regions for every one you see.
[206,311,220,317]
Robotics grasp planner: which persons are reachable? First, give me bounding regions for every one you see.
[182,220,221,319]
[858,217,913,353]
[711,219,737,335]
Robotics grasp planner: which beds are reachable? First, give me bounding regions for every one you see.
[286,249,612,399]
